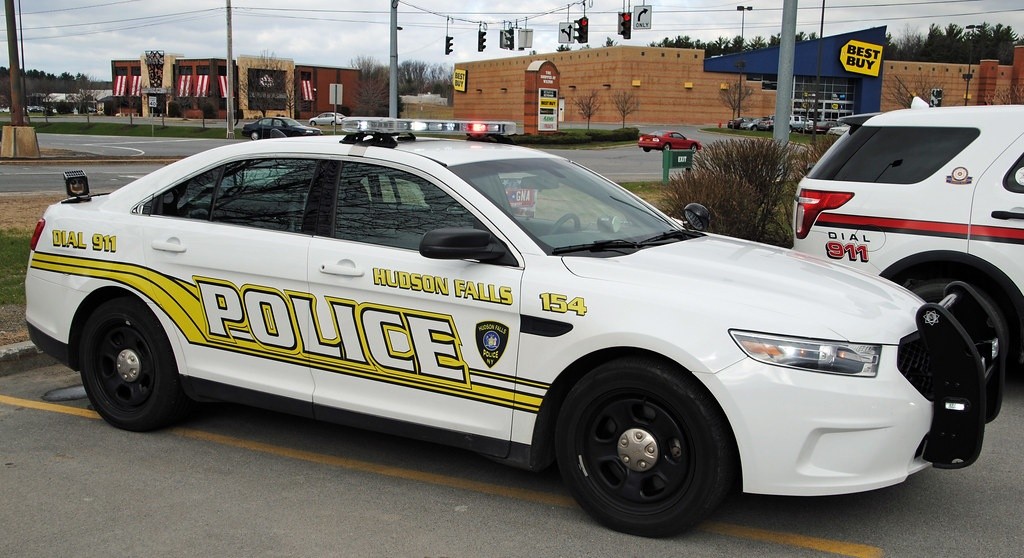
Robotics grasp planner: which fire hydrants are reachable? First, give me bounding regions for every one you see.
[717,121,723,128]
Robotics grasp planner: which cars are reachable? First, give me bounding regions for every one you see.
[637,131,702,153]
[728,115,850,136]
[24,115,1009,540]
[309,112,345,126]
[239,114,322,140]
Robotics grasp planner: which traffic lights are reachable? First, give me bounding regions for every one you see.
[445,36,453,56]
[504,28,514,51]
[619,12,632,40]
[478,30,486,53]
[573,15,589,44]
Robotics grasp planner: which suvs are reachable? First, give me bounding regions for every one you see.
[790,97,1022,372]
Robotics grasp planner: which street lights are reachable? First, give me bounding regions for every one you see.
[961,24,986,106]
[735,5,753,117]
